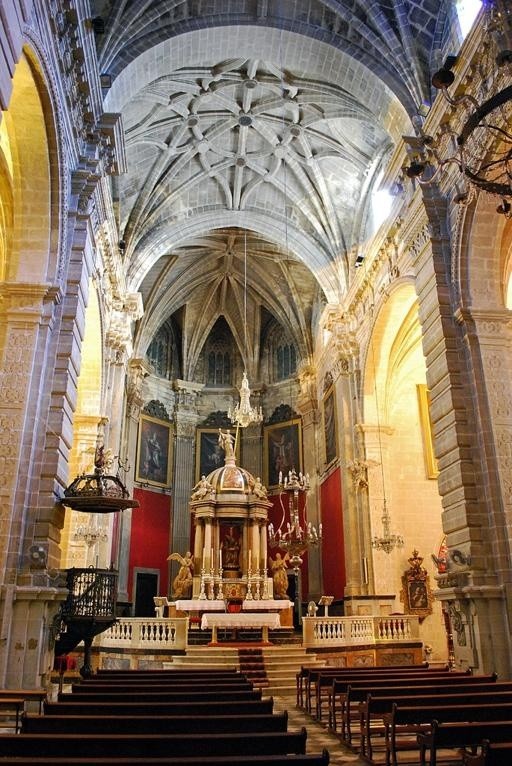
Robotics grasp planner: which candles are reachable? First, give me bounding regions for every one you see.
[202,547,223,569]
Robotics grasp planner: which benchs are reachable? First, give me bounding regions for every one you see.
[0,667,330,766]
[294,657,512,766]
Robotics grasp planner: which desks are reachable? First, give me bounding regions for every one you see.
[202,614,282,644]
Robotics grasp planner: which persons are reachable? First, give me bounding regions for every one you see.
[219,427,236,458]
[270,433,292,477]
[270,552,288,598]
[172,552,194,599]
[139,431,163,482]
[203,435,221,464]
[250,477,267,499]
[190,475,213,502]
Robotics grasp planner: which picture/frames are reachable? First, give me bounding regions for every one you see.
[399,567,435,621]
[318,385,339,477]
[136,413,176,490]
[194,425,242,486]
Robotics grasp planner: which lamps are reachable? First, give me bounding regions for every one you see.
[245,548,269,583]
[267,466,323,578]
[398,0,512,220]
[227,372,264,428]
[369,307,405,555]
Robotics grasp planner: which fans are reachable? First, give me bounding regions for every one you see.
[27,544,48,566]
[447,548,470,567]
